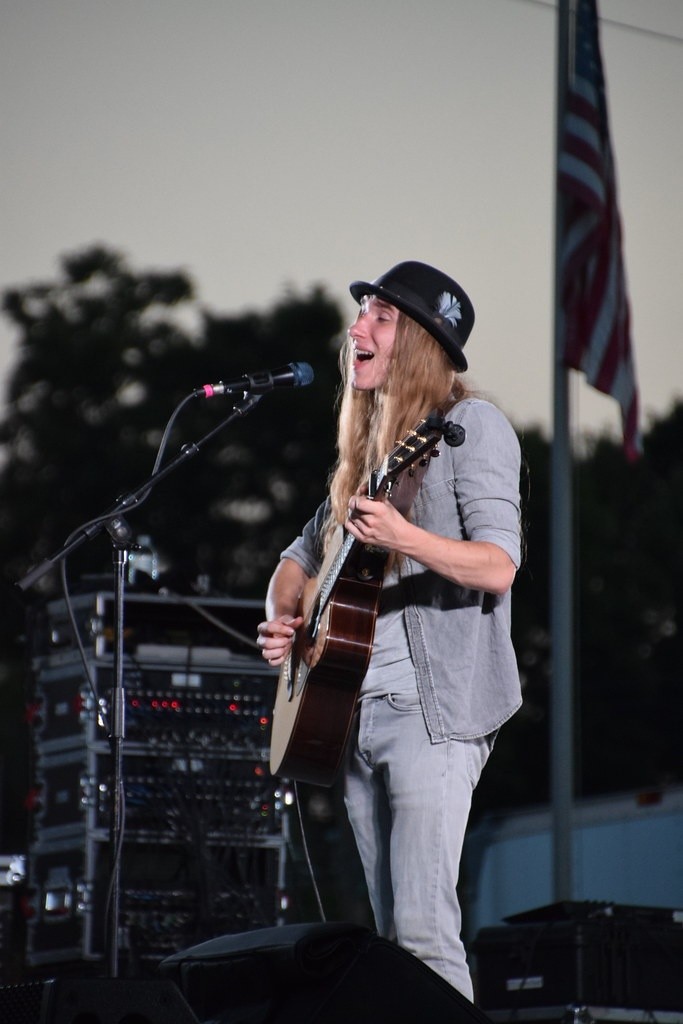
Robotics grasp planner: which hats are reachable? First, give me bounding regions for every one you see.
[348,261,474,372]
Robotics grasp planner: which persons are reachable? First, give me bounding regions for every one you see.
[255,260,524,1003]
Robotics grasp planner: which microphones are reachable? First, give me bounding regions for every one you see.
[195,361,314,398]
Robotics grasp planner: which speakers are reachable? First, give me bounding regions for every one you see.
[164,919,489,1023]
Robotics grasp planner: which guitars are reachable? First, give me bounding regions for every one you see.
[269,408,466,786]
